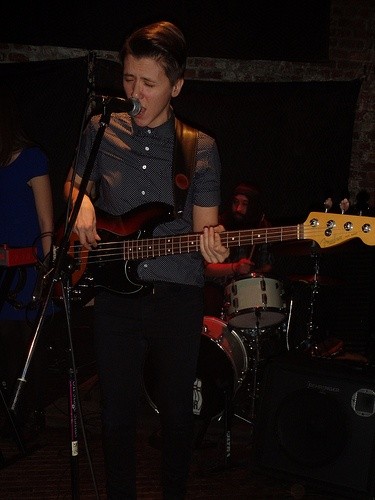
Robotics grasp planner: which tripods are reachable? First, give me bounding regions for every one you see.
[0,247,254,469]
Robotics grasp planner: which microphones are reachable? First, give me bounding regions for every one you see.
[92,95,142,117]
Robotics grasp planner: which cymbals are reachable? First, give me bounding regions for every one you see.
[288,275,349,289]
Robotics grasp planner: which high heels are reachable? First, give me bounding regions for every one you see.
[19,401,47,440]
[0,400,22,440]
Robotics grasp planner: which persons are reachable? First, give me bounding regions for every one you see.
[202,181,280,287]
[64,21,231,500]
[0,86,57,433]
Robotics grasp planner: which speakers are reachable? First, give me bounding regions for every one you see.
[251,356,375,500]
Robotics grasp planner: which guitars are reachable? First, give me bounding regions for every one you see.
[48,197,375,302]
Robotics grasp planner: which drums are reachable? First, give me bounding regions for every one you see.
[143,316,248,421]
[220,273,290,331]
